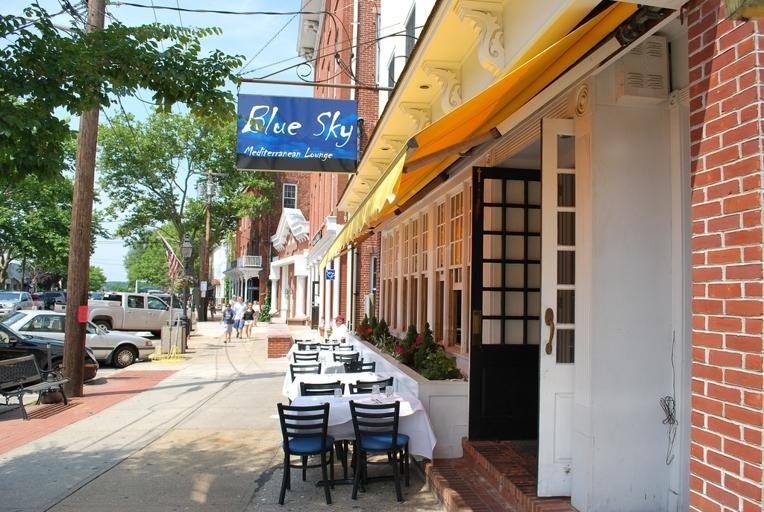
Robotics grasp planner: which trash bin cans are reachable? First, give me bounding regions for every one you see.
[161,320,186,354]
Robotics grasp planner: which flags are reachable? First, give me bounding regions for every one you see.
[161,236,179,279]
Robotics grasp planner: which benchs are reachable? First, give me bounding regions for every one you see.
[0,353,71,420]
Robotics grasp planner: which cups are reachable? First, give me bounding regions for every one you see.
[371,384,394,402]
[333,387,343,399]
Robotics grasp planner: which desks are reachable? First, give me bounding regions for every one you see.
[287,392,436,485]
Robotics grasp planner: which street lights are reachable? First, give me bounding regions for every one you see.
[135,280,147,292]
[181,232,194,317]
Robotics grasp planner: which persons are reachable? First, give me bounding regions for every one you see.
[209,295,260,343]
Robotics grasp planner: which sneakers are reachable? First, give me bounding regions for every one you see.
[223,332,252,344]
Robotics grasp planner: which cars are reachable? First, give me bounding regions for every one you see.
[0,287,195,381]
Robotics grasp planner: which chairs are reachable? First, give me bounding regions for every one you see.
[276,338,409,505]
